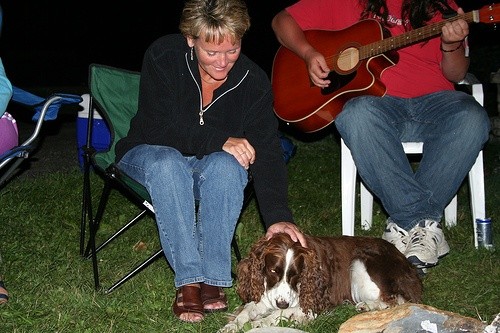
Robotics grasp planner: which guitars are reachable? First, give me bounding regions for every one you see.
[271,2,500,133]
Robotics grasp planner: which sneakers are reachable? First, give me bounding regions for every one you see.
[403,218,450,268]
[381,217,409,254]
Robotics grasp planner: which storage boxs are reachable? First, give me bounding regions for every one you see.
[77,94,110,165]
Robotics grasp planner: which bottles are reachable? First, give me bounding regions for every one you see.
[477,218,491,248]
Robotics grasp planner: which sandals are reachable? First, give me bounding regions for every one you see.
[199,282,229,315]
[173,285,206,324]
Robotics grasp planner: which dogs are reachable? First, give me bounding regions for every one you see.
[214,230,427,332]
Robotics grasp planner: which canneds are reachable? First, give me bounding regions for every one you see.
[476,218,493,251]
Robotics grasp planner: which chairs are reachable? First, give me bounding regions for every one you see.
[340,75,487,249]
[0,84,83,186]
[80,62,297,294]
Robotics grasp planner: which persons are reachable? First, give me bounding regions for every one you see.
[272,0,492,275]
[114,0,307,322]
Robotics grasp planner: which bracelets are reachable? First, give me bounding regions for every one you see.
[440,43,463,52]
